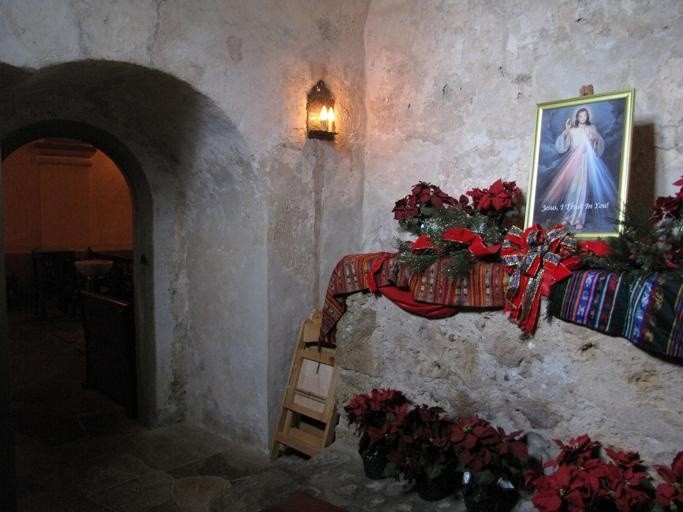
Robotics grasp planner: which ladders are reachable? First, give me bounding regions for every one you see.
[268,309,343,466]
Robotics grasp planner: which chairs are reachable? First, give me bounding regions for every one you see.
[32,247,134,419]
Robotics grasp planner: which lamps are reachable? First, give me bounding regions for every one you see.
[305,80,337,138]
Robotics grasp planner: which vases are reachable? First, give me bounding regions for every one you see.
[464,485,520,511]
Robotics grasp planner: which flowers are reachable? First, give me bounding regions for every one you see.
[531,431,682,511]
[345,386,543,502]
[652,177,683,267]
[390,179,523,251]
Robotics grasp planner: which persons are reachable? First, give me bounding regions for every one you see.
[554,106,606,230]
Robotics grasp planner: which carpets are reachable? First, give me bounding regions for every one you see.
[259,490,345,511]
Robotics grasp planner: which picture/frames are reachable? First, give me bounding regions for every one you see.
[523,88,636,242]
